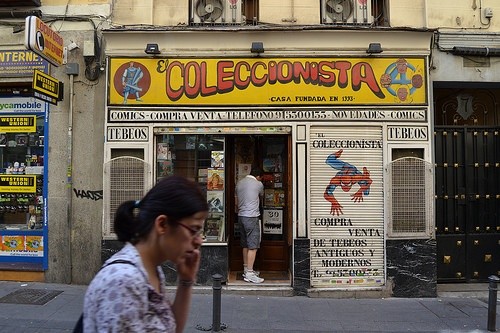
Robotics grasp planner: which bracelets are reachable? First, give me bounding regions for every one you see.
[178,279,194,288]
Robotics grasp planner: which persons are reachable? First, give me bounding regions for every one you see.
[234,166,265,283]
[83,176,209,333]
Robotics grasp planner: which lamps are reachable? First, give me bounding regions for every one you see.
[368,43,383,54]
[251,42,264,55]
[145,44,161,56]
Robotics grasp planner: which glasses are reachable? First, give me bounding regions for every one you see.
[175,222,207,240]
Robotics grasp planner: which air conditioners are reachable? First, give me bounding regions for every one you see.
[192,0,242,23]
[321,0,372,23]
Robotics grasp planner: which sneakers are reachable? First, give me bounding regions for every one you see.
[244,270,264,284]
[242,267,260,277]
